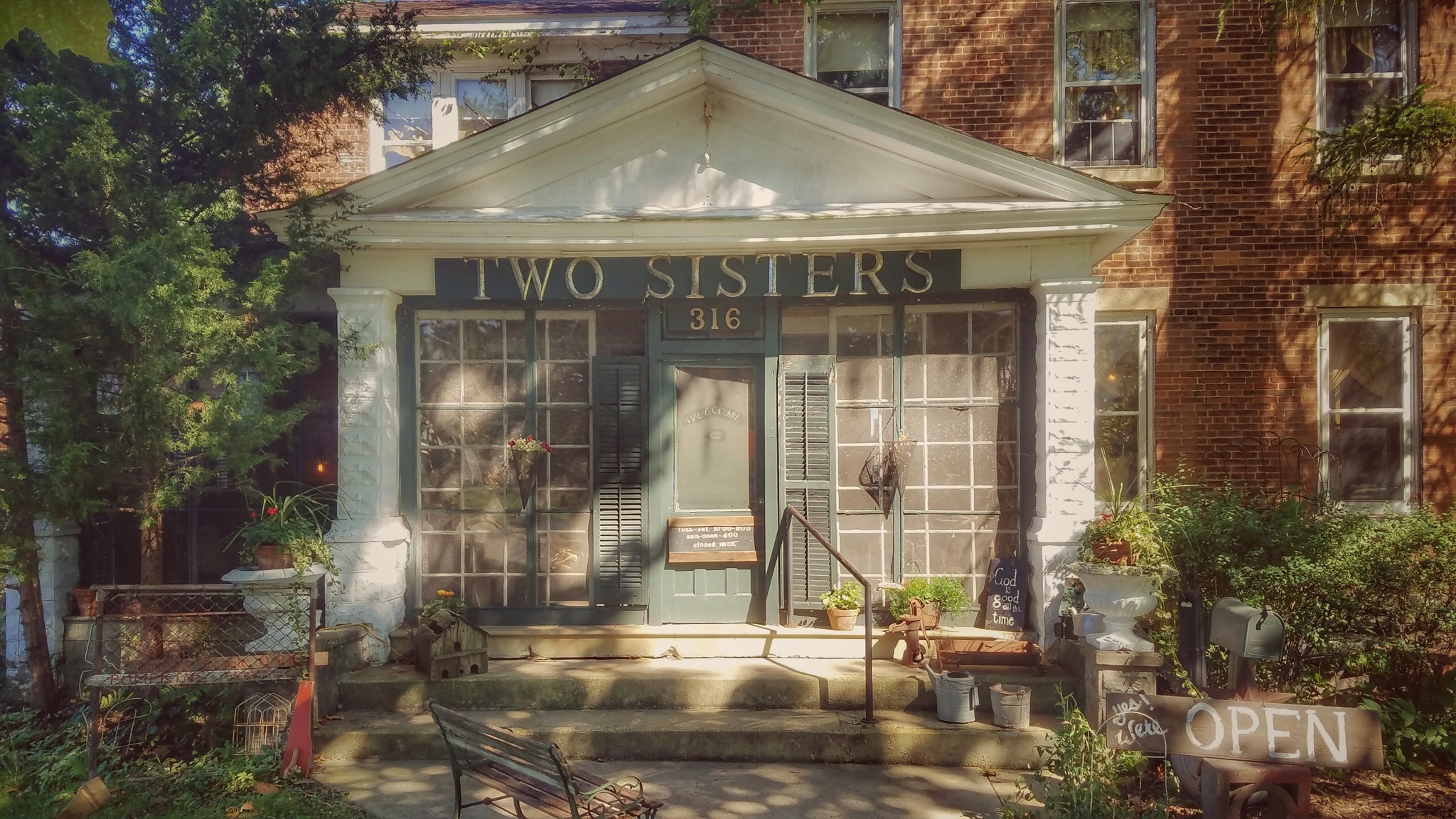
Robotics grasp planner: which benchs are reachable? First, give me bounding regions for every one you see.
[78,582,328,783]
[424,698,663,819]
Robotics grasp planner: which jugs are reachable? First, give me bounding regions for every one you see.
[918,658,981,723]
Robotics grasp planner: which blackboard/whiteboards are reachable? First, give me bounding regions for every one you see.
[983,556,1030,633]
[666,516,766,561]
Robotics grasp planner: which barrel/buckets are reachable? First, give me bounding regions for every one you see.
[989,682,1033,729]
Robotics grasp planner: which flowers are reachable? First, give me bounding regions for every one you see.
[1073,442,1206,699]
[420,590,467,616]
[216,480,356,682]
[509,435,553,479]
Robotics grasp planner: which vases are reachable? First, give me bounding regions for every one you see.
[511,449,544,510]
[416,615,436,629]
[935,638,1044,678]
[253,543,295,570]
[1086,539,1134,565]
[75,586,98,617]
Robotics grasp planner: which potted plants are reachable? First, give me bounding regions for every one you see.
[883,429,919,502]
[881,572,975,630]
[821,581,862,632]
